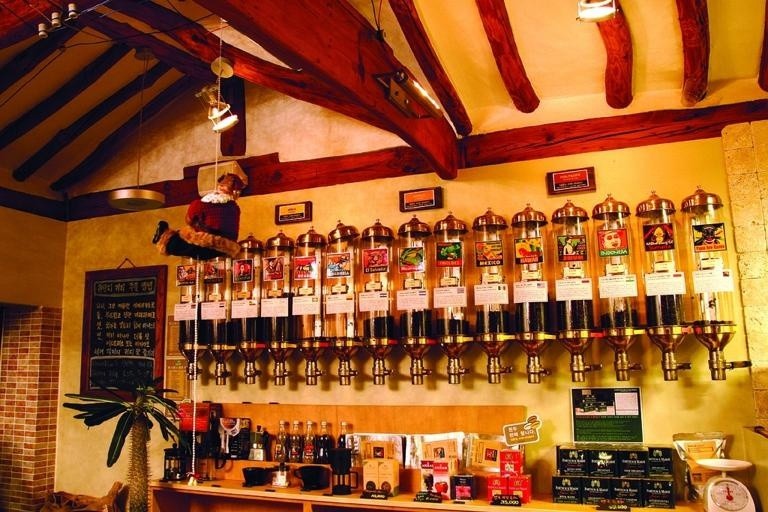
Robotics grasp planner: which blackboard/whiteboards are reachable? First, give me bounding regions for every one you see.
[81,265,167,402]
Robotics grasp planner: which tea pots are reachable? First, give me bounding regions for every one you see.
[330,447,359,493]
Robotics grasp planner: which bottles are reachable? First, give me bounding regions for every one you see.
[204,409,220,454]
[172,187,733,388]
[274,420,348,462]
[163,443,187,479]
[247,425,266,460]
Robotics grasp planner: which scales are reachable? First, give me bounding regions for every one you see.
[696,459,756,512]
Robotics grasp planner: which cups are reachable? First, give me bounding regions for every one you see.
[243,467,263,482]
[295,464,328,488]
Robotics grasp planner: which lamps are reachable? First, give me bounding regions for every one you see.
[199,16,234,205]
[195,55,241,134]
[38,3,78,38]
[107,49,166,209]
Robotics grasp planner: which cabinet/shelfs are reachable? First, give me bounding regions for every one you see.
[146,458,704,512]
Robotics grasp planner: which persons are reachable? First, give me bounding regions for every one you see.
[152,174,243,262]
